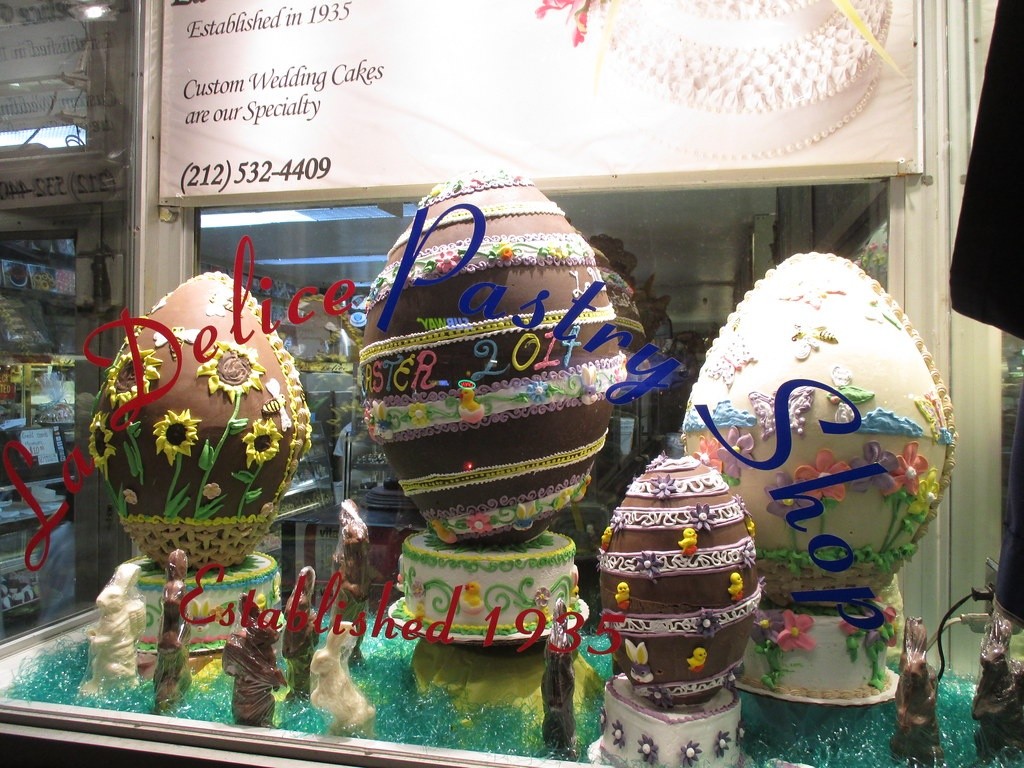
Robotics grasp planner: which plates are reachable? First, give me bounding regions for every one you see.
[9,274,28,287]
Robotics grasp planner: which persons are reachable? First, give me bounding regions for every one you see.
[333,422,352,482]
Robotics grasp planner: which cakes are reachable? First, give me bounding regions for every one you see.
[565,0,894,156]
[90,166,960,768]
